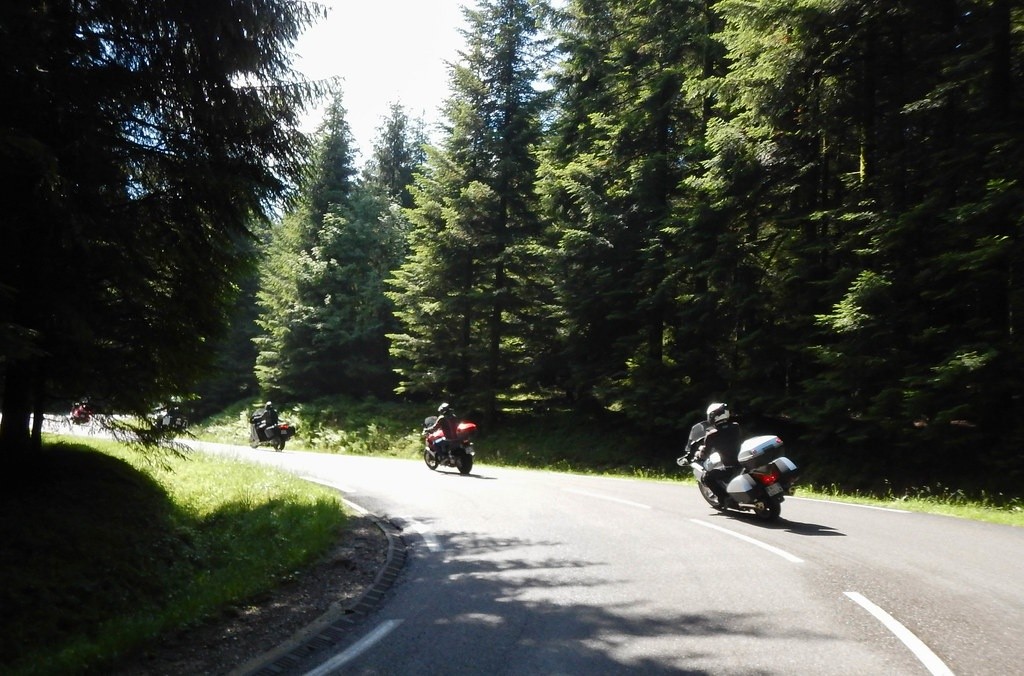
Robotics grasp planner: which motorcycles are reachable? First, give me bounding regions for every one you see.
[677,420,800,525]
[420,416,476,474]
[247,408,295,452]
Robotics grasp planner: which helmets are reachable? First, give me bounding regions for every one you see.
[706,402,729,426]
[438,403,449,411]
[265,401,273,409]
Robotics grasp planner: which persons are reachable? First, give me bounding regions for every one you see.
[697,403,742,509]
[424,403,459,465]
[254,402,278,441]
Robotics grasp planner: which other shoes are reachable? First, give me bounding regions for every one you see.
[440,458,448,465]
[718,496,732,507]
